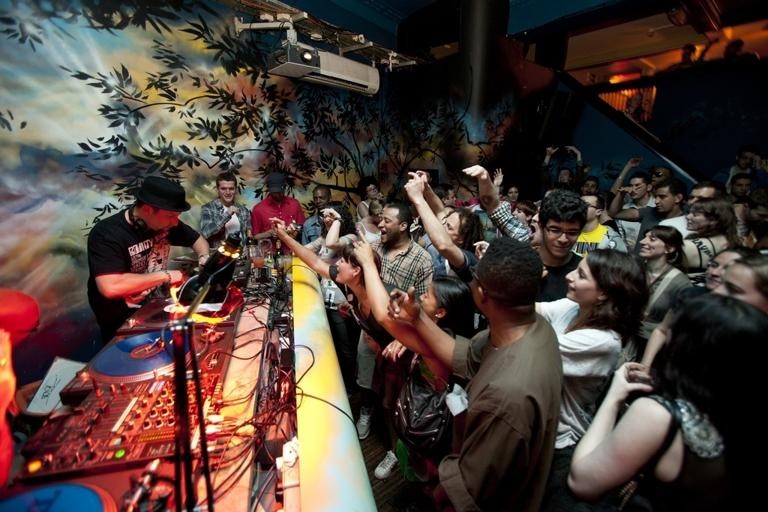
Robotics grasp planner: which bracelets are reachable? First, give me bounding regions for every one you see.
[157,270,171,289]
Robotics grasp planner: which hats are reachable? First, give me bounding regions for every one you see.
[268,173,284,192]
[132,176,191,211]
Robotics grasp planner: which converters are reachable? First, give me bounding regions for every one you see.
[280,348,292,370]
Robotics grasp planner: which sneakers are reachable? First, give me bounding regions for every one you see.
[356,408,372,439]
[374,450,399,479]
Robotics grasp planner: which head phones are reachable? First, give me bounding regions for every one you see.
[128,205,147,232]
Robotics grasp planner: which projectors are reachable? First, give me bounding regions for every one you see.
[267,43,320,79]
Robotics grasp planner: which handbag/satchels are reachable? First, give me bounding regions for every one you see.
[391,376,450,451]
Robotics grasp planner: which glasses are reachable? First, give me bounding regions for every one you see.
[544,225,581,238]
[653,171,664,178]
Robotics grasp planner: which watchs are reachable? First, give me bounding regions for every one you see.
[196,253,210,259]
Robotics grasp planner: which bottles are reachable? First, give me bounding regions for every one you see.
[245,226,255,245]
[274,240,284,258]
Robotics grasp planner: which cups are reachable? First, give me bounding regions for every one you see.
[249,239,273,267]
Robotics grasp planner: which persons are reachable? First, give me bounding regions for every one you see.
[250,172,305,254]
[723,38,758,57]
[269,145,767,511]
[199,173,252,248]
[677,36,720,62]
[622,92,644,122]
[87,176,211,345]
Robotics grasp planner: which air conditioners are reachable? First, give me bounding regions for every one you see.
[300,47,380,97]
